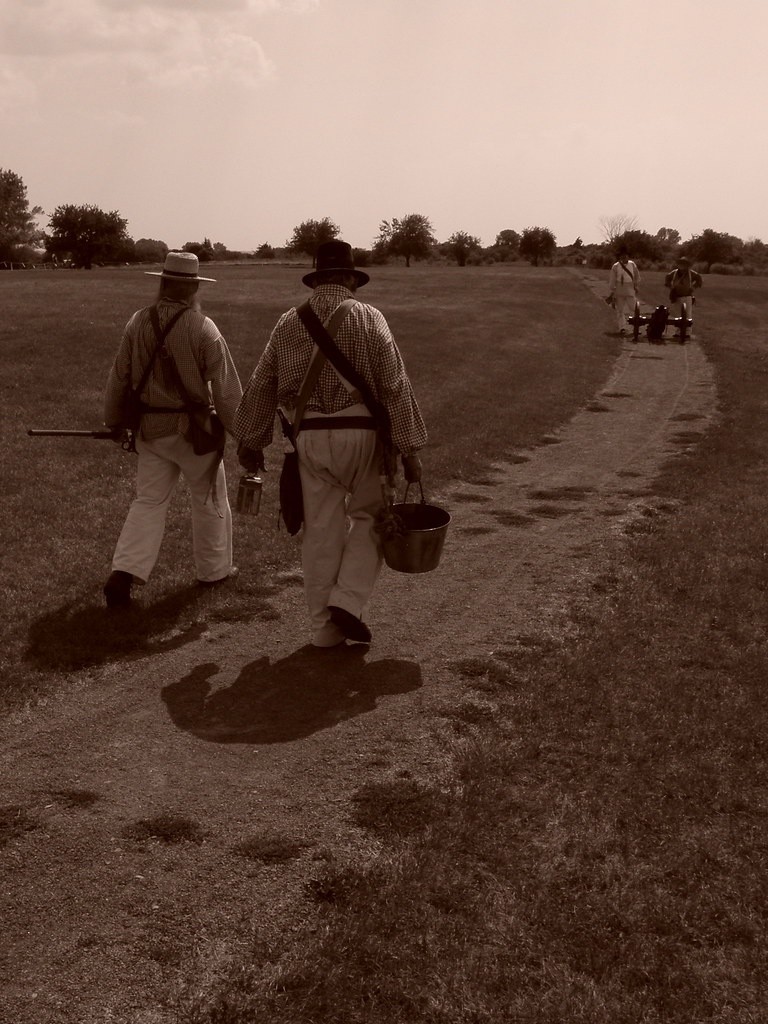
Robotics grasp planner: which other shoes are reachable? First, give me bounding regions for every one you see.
[330,607,372,643]
[310,631,346,648]
[196,565,239,585]
[102,570,134,608]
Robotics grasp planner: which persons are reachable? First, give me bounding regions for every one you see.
[664,256,702,338]
[231,241,430,652]
[609,248,642,335]
[103,252,243,610]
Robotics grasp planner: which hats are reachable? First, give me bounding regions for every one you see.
[144,251,217,282]
[615,245,630,257]
[675,256,692,267]
[300,241,370,288]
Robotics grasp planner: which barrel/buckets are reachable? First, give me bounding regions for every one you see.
[236,476,264,516]
[377,503,452,573]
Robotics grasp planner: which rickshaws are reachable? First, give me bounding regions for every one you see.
[628,302,693,344]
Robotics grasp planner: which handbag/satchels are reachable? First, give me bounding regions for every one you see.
[277,450,304,537]
[669,287,678,303]
